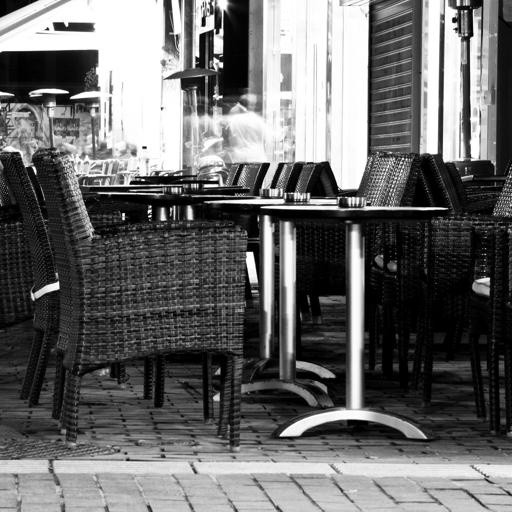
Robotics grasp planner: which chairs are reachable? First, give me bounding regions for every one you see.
[0,148,510,451]
